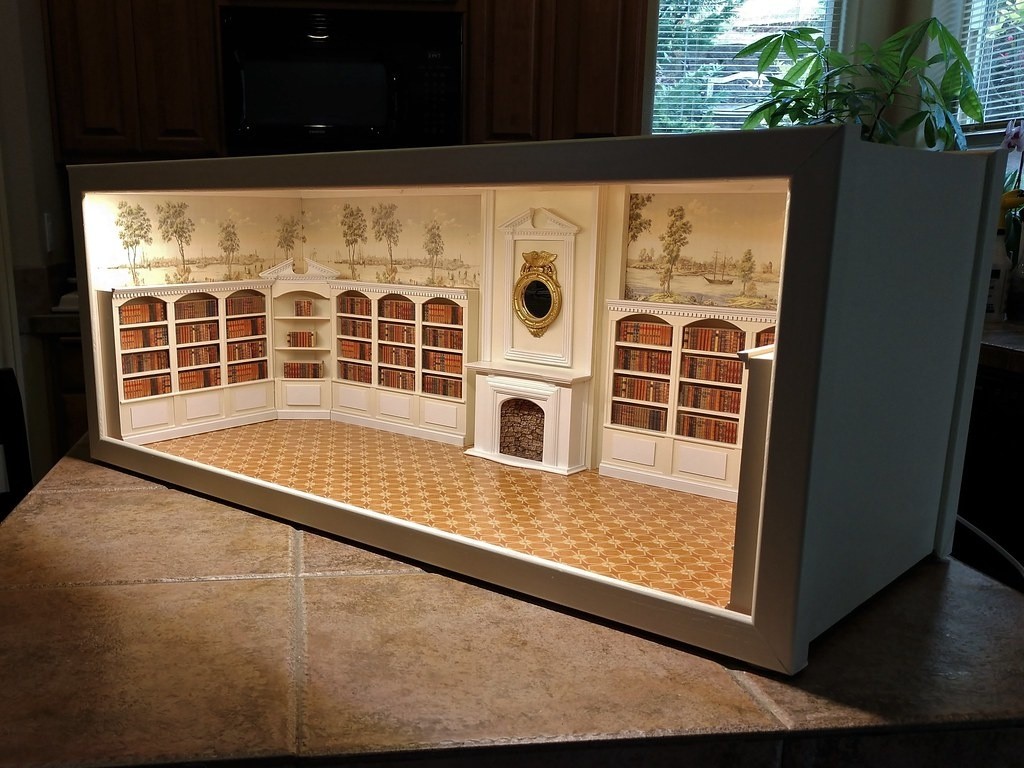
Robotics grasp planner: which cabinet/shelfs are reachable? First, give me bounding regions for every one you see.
[270,278,332,420]
[97,279,278,447]
[598,300,777,505]
[40,0,221,164]
[32,311,89,463]
[332,279,480,448]
[467,0,648,145]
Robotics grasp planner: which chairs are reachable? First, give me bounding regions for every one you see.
[0,367,32,525]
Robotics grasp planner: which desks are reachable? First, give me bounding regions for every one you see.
[1,426,1024,768]
[955,321,1024,594]
